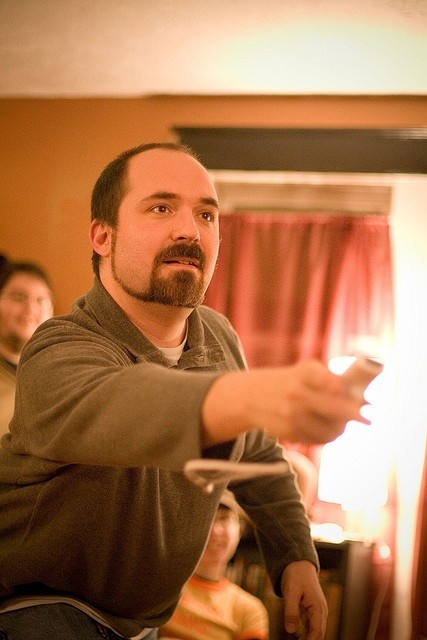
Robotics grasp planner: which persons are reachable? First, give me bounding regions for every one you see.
[161,489,270,640]
[1,263,54,435]
[1,143,370,640]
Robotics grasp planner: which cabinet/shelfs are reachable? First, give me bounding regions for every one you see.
[224,538,371,638]
